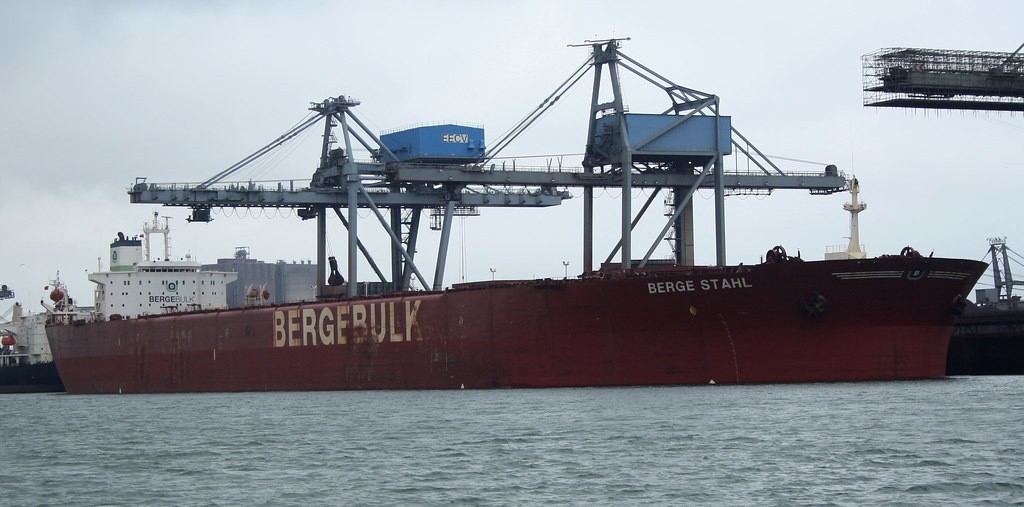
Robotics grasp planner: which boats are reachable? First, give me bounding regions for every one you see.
[943,290,1024,374]
[0,301,68,395]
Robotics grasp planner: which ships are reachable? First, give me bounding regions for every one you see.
[42,33,993,396]
